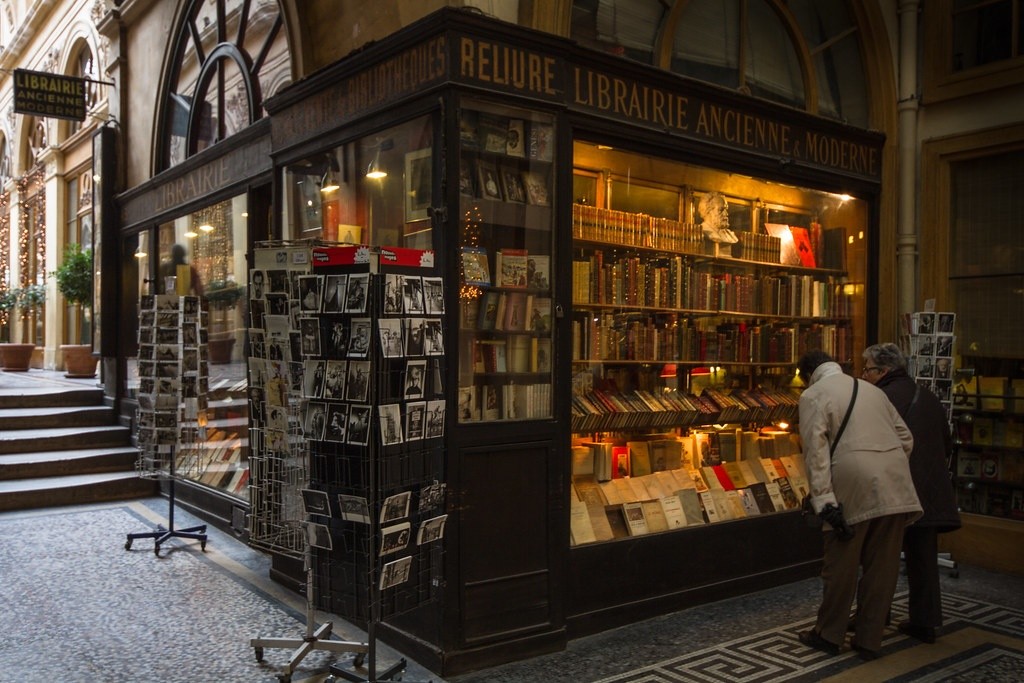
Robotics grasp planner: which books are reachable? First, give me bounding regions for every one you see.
[136,294,208,445]
[458,245,552,423]
[456,116,551,206]
[175,428,249,494]
[911,311,955,421]
[250,271,448,590]
[572,204,851,546]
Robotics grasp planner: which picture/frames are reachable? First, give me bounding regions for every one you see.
[245,245,449,590]
[138,293,205,441]
[500,164,526,205]
[900,308,954,430]
[404,148,431,223]
[477,160,502,201]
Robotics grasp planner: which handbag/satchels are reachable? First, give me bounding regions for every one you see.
[799,496,813,526]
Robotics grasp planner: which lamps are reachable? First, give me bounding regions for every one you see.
[366,145,387,179]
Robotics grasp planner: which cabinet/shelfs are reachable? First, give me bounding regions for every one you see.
[476,285,551,386]
[573,237,849,366]
[945,406,1024,523]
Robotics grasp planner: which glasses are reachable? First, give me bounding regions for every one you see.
[862,366,883,375]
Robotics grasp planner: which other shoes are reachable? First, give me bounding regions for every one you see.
[799,628,840,654]
[850,634,876,661]
[898,619,937,643]
[846,611,892,630]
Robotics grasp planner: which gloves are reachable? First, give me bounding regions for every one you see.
[819,502,856,542]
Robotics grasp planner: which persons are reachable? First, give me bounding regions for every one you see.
[269,338,284,360]
[315,365,323,386]
[697,191,738,244]
[921,358,933,377]
[251,271,265,300]
[936,359,948,379]
[328,281,340,308]
[919,316,932,333]
[795,350,924,656]
[405,368,421,395]
[861,342,961,644]
[921,337,933,355]
[159,245,200,295]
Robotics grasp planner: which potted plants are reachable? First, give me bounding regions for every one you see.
[206,279,243,363]
[0,284,46,368]
[56,248,98,375]
